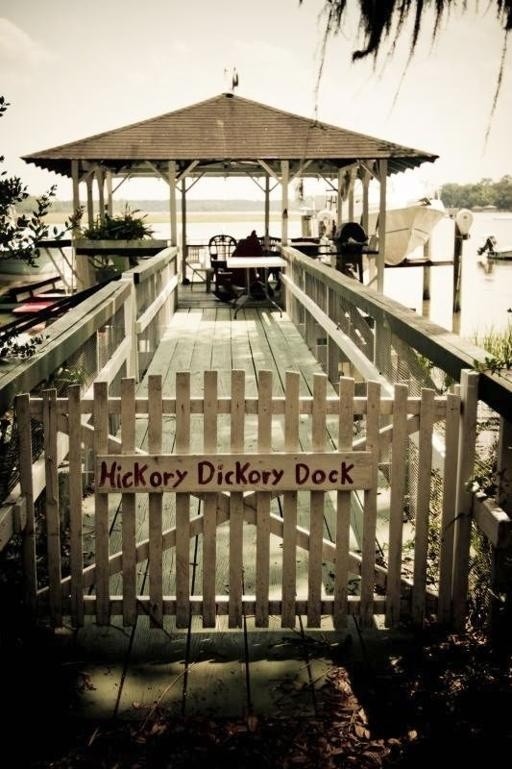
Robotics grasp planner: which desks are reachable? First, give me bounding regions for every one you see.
[226,256,286,321]
[259,241,318,250]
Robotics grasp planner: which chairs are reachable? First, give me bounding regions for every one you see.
[209,235,240,289]
